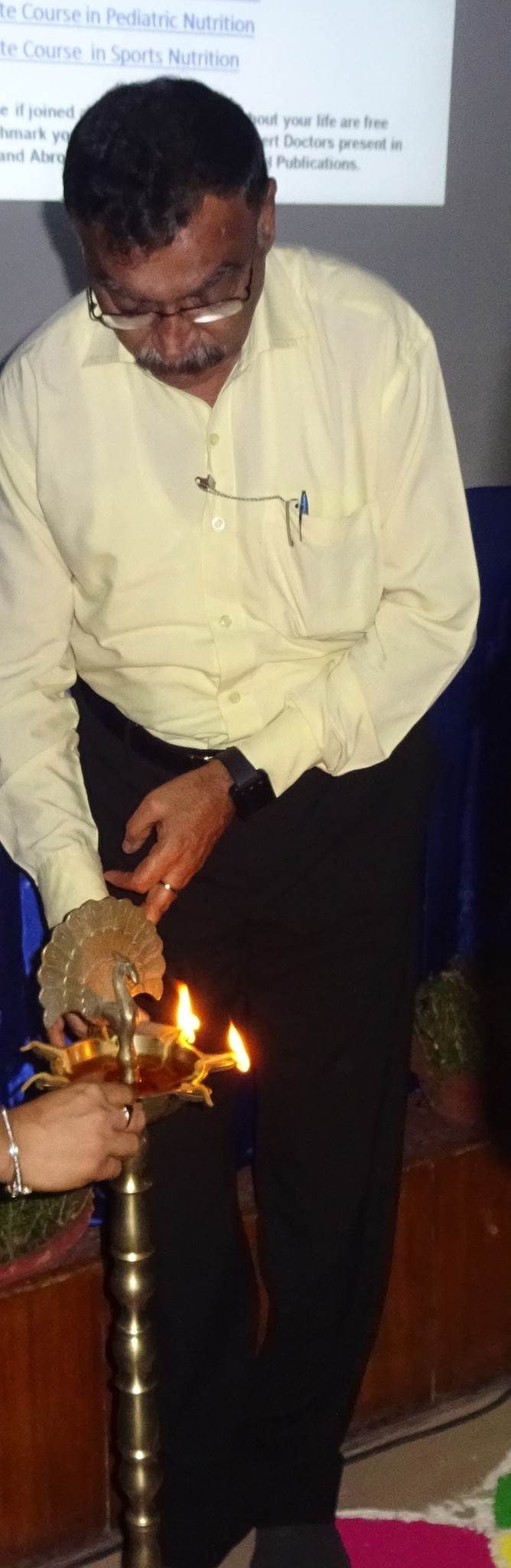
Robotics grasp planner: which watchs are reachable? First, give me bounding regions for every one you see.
[219,746,278,822]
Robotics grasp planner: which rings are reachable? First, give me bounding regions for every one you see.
[120,1104,134,1129]
[159,880,183,896]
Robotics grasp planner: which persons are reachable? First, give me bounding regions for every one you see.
[0,78,485,1568]
[1,1082,146,1191]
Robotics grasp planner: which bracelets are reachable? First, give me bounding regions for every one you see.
[0,1105,32,1198]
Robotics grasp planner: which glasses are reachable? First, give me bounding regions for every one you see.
[87,256,252,333]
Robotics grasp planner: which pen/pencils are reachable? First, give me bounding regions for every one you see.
[299,490,308,542]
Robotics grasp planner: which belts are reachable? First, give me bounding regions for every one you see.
[116,715,218,773]
